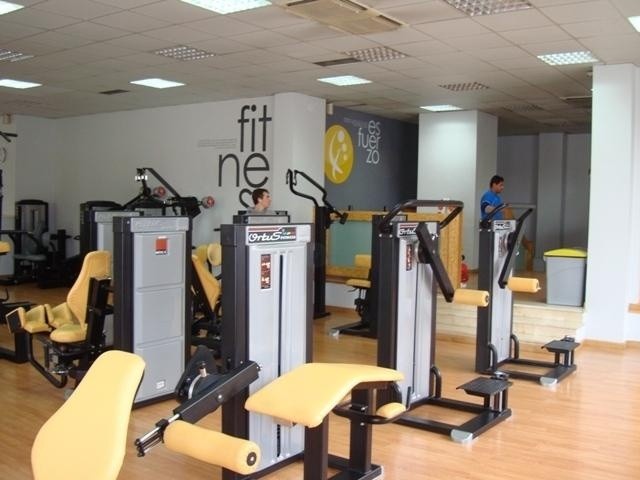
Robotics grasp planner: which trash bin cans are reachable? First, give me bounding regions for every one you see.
[543,247,587,306]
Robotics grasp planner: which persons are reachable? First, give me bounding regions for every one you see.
[247,188,273,215]
[479,175,512,221]
